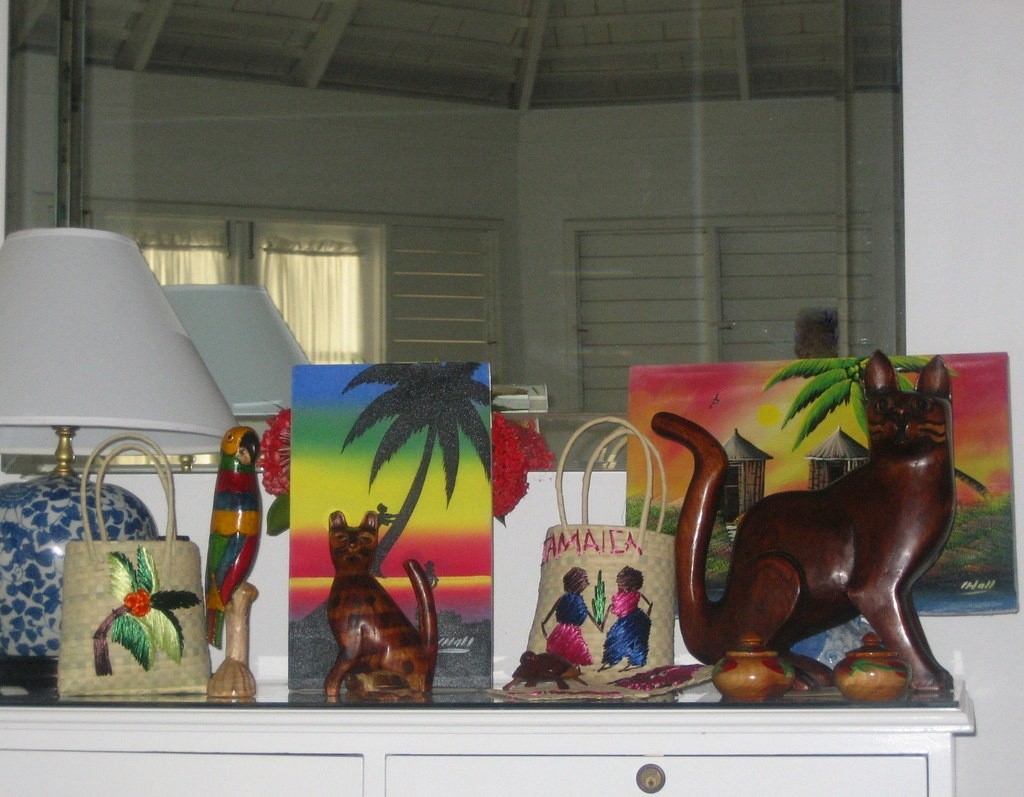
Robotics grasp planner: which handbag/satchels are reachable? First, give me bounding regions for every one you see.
[525,416,676,699]
[59,432,212,700]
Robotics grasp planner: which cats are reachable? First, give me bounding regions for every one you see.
[322,511,440,698]
[648,349,960,695]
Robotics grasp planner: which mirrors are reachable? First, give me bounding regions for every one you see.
[0,0,901,467]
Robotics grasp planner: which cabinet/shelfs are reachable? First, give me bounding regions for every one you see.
[0,681,981,797]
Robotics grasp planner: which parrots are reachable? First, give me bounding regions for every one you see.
[203,425,262,651]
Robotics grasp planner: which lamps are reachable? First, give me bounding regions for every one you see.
[153,277,314,470]
[0,229,239,662]
